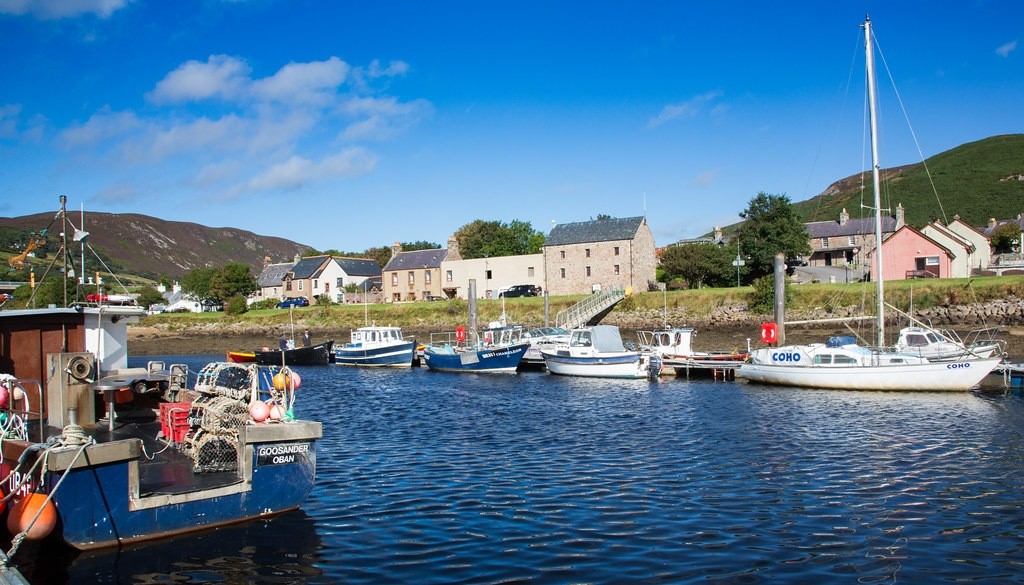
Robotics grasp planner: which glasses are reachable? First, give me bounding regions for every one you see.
[306,332,308,333]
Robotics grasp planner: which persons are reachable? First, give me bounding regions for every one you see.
[302,329,312,347]
[279,332,289,350]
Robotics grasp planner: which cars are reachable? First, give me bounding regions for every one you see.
[276,296,309,308]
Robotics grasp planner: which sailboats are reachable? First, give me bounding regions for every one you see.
[734,10,1008,391]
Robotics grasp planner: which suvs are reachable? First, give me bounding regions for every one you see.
[499,284,543,299]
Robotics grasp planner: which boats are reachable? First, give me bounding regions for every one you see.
[228,352,255,363]
[331,325,419,367]
[630,325,749,363]
[0,194,323,552]
[539,325,664,379]
[328,341,427,366]
[421,322,532,375]
[505,326,571,364]
[255,337,335,367]
